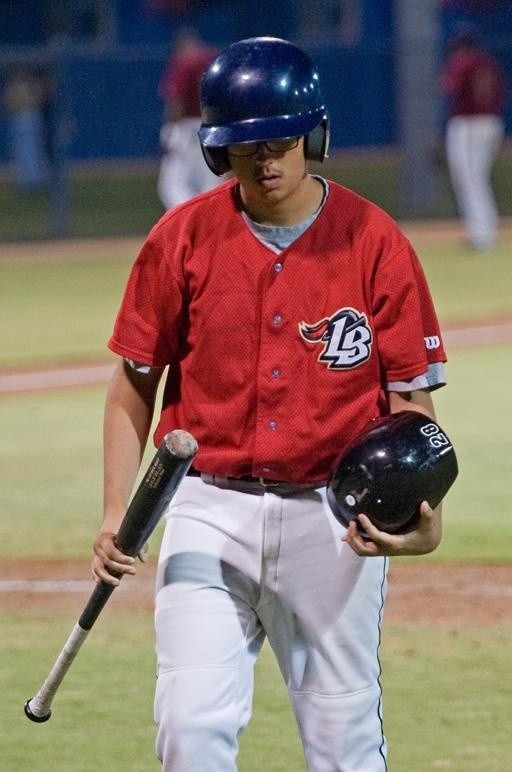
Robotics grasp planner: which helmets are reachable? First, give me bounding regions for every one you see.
[197,36,330,177]
[325,410,459,542]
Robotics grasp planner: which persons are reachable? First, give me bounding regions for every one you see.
[89,33,456,770]
[440,36,506,250]
[158,25,228,208]
[4,64,76,236]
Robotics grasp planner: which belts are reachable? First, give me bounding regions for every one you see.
[189,467,335,499]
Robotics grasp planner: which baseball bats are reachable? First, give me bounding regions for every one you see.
[24,428,198,723]
